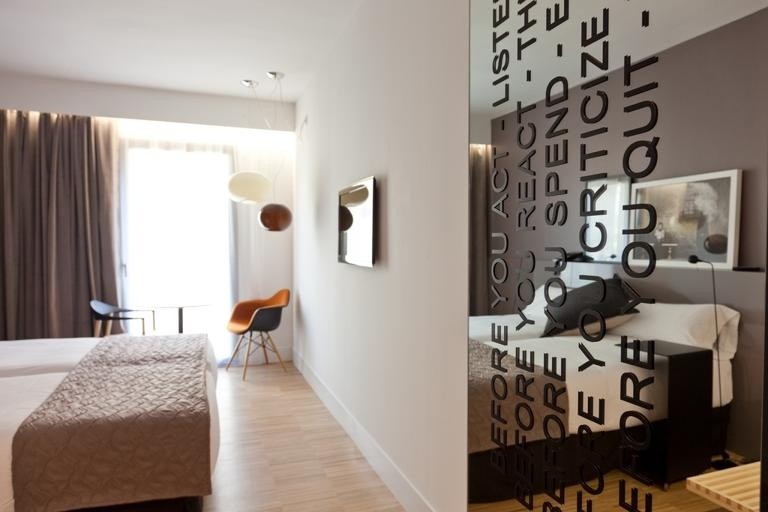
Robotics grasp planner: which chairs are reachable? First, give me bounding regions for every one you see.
[87,288,291,382]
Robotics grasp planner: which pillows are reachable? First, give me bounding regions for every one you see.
[541,275,641,338]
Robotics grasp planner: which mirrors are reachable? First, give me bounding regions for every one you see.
[468,0,766,512]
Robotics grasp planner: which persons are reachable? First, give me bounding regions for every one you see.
[652,222,666,259]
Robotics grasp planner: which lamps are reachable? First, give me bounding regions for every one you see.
[227,71,308,234]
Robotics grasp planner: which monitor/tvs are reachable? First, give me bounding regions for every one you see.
[337,176,374,269]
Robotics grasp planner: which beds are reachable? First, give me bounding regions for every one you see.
[0,333,221,512]
[467,283,742,503]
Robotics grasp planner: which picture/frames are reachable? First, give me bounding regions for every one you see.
[583,169,744,271]
